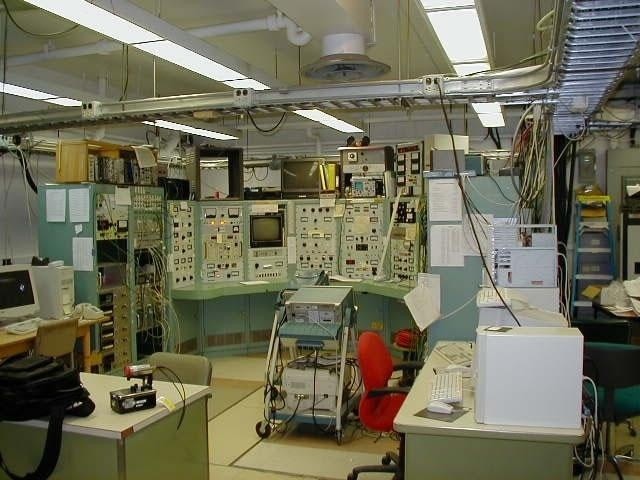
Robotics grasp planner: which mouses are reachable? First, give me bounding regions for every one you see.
[427,401,453,414]
[445,364,467,372]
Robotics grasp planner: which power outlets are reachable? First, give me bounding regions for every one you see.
[423,74,444,96]
[232,88,254,110]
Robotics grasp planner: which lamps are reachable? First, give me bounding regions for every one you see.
[1,82,238,140]
[28,2,362,135]
[421,0,507,128]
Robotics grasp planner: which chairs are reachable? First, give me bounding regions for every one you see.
[348,331,425,480]
[147,351,212,386]
[2,316,79,376]
[583,342,640,480]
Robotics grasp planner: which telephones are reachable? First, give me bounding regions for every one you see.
[72,303,104,320]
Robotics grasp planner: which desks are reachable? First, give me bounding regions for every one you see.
[0,315,110,374]
[393,339,586,479]
[0,372,213,480]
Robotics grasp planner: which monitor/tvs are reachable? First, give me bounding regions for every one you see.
[478,298,568,327]
[0,264,40,321]
[281,158,325,198]
[250,215,283,248]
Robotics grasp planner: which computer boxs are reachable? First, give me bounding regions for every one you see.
[32,266,74,319]
[474,326,584,429]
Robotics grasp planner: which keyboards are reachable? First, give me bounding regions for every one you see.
[431,372,462,403]
[434,341,472,366]
[7,318,43,335]
[476,286,512,308]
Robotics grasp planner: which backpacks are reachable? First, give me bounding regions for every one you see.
[0,352,95,480]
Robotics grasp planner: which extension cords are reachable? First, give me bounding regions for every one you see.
[296,340,324,351]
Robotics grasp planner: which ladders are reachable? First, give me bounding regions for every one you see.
[570,194,617,321]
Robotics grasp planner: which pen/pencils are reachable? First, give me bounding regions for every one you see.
[433,368,437,376]
